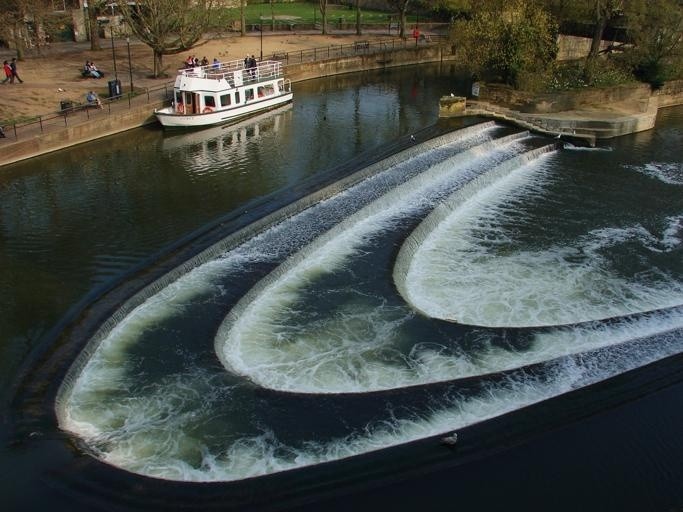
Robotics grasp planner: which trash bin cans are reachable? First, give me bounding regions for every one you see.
[108,79,122,100]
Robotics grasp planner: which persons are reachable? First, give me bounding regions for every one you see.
[202,56,209,69]
[192,55,199,67]
[212,58,222,70]
[1,61,12,84]
[10,58,24,83]
[87,91,102,108]
[250,55,256,78]
[410,25,429,44]
[84,61,101,78]
[244,55,251,73]
[185,56,193,68]
[90,62,101,79]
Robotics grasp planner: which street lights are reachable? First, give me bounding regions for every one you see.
[260,14,264,61]
[126,34,133,92]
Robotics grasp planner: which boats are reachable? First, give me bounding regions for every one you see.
[154,59,293,132]
